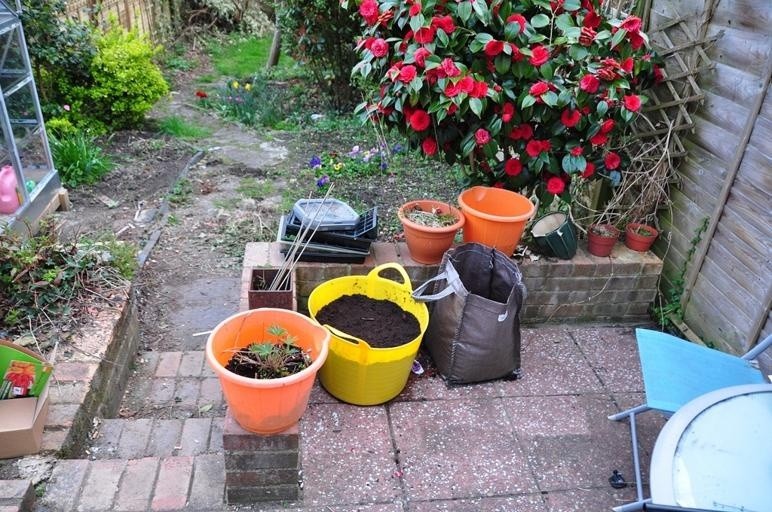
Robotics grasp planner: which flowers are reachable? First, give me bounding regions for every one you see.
[408,206,458,228]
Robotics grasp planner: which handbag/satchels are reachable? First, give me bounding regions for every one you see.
[411,243,526,387]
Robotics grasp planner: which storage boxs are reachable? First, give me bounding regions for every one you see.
[0,339,60,460]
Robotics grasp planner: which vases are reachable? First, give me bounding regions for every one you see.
[398,200,465,265]
[531,211,577,260]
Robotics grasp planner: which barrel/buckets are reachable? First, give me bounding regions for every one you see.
[1,165,19,214]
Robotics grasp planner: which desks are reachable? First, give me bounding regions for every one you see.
[649,384,772,512]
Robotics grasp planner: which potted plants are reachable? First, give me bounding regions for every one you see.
[206,307,330,434]
[588,224,620,256]
[625,224,659,252]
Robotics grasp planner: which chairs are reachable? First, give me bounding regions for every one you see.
[608,328,772,512]
